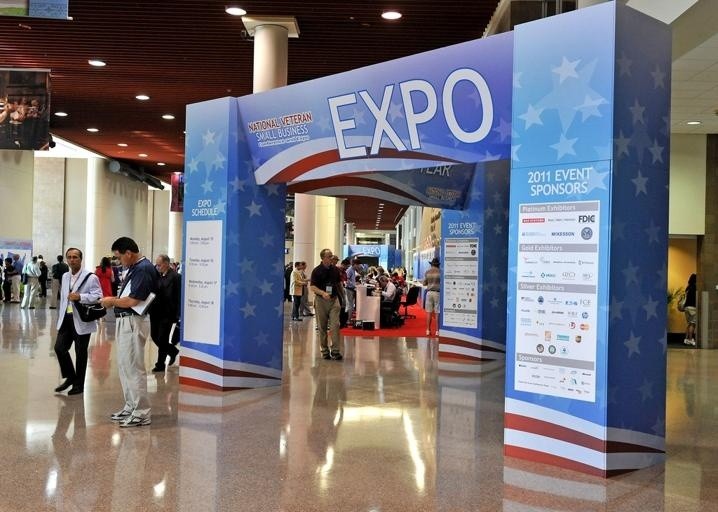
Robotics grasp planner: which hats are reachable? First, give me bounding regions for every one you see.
[428,256,441,266]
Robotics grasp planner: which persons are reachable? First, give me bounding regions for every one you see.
[684,273,697,346]
[0,236,182,427]
[0,96,47,141]
[284,246,442,361]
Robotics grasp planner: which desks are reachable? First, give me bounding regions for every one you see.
[356,284,381,329]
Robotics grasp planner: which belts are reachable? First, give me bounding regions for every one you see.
[113,311,149,318]
[427,289,441,292]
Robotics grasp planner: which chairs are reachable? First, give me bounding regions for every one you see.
[380,289,404,327]
[400,287,419,318]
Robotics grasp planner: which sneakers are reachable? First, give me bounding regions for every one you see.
[426,330,431,336]
[119,414,152,428]
[435,330,439,336]
[110,409,132,421]
[323,355,331,360]
[330,351,343,360]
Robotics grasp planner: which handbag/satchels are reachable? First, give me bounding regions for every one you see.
[74,272,108,323]
[20,273,28,284]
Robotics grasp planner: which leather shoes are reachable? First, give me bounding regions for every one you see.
[169,350,179,366]
[49,306,57,309]
[68,386,85,395]
[29,306,35,309]
[152,364,166,373]
[292,318,303,321]
[54,377,75,393]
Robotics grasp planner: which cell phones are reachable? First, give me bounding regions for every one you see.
[96,297,101,300]
